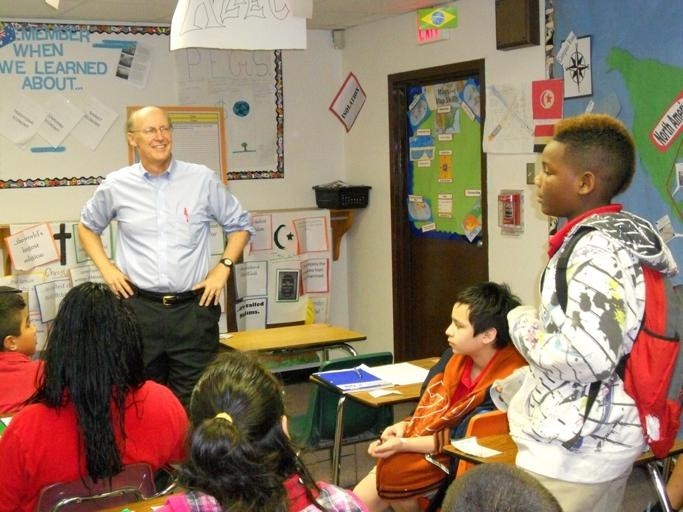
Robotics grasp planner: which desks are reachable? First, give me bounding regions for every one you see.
[219,323,683,511]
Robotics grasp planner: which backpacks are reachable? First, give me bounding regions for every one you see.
[555,226,682,459]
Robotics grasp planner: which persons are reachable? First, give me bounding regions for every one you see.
[76,107,256,416]
[439,461,564,512]
[0,283,47,417]
[489,112,680,512]
[350,279,530,510]
[1,279,191,511]
[158,348,374,512]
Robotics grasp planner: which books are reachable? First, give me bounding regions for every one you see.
[310,361,394,395]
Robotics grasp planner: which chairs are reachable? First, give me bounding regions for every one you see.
[37,463,157,512]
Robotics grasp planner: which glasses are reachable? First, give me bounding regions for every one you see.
[128,126,169,135]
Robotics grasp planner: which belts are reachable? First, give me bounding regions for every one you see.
[125,280,204,307]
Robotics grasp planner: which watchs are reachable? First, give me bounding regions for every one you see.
[220,257,234,269]
